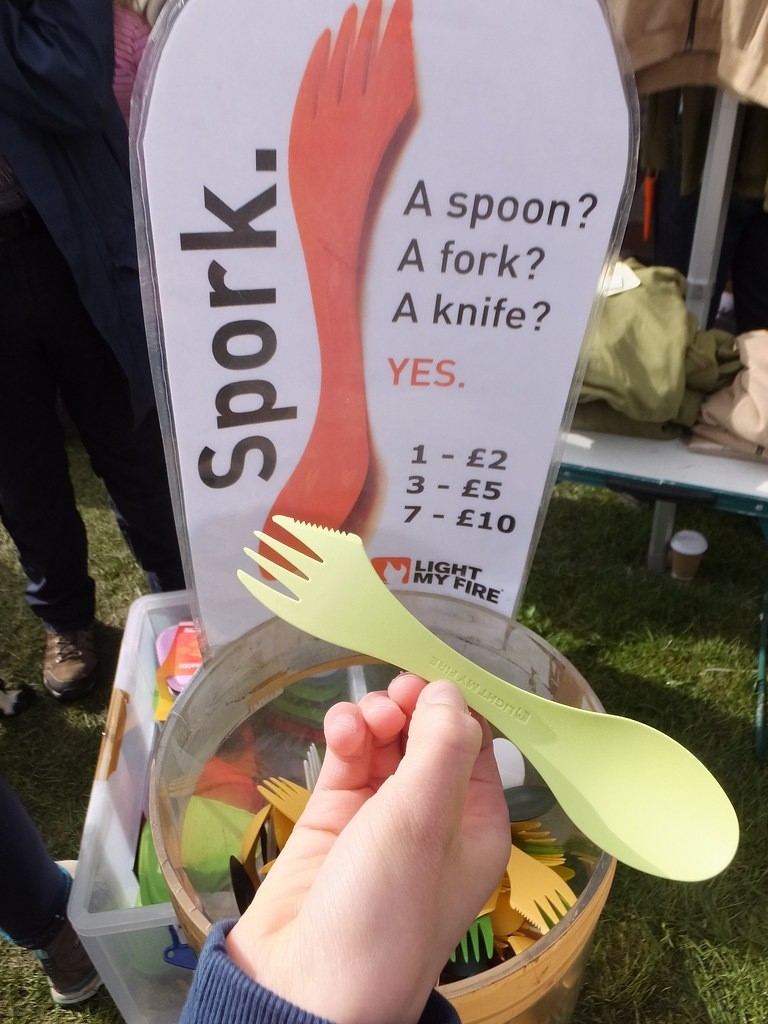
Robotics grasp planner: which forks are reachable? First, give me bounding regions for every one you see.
[238,514,741,883]
[257,0,416,581]
[259,744,578,963]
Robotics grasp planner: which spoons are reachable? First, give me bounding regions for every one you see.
[240,804,273,889]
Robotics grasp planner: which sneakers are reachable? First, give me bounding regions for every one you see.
[32,872,130,1008]
[42,623,101,701]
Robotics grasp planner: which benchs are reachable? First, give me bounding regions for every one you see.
[558,430,768,519]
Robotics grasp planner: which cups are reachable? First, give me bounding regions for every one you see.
[670,530,708,580]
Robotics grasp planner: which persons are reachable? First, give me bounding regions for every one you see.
[0,774,108,1008]
[176,670,511,1024]
[602,0,767,327]
[1,0,190,699]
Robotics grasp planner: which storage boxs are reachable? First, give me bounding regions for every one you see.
[66,592,238,1024]
